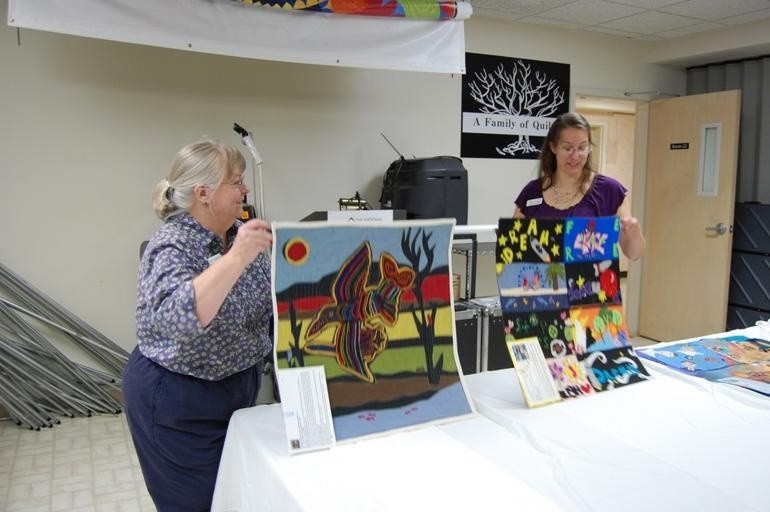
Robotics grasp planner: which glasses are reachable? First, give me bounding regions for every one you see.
[555,142,594,153]
[218,175,246,190]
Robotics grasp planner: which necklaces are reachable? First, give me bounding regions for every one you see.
[551,182,582,205]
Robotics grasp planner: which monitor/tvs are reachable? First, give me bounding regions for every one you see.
[384,154,468,226]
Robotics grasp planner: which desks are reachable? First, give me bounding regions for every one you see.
[229,326,768,511]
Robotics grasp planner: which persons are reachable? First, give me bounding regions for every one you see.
[121,135,274,512]
[512,111,647,262]
